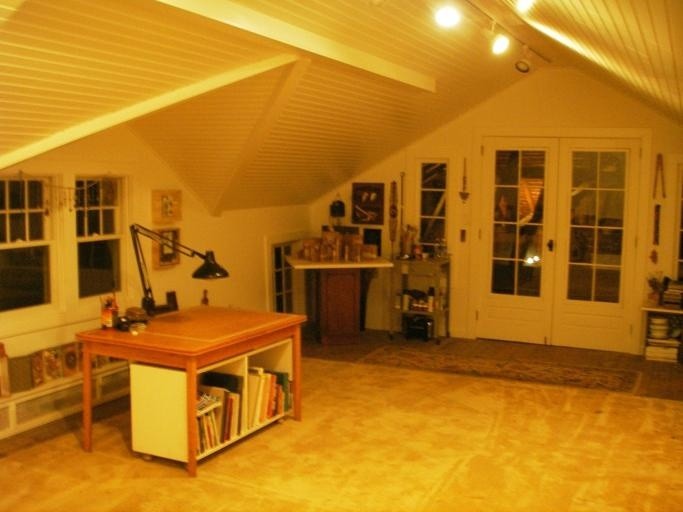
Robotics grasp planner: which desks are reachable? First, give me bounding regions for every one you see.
[75,305,308,477]
[284,255,392,339]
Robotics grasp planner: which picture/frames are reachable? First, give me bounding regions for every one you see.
[351,182,384,225]
[151,189,183,226]
[152,229,181,269]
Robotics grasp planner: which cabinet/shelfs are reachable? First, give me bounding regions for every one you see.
[130,338,294,462]
[387,257,450,344]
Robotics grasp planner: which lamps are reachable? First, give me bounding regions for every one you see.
[483,20,509,54]
[516,42,531,72]
[130,223,228,316]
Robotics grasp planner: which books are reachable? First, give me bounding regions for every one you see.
[192,371,243,455]
[247,365,286,427]
[664,281,683,304]
